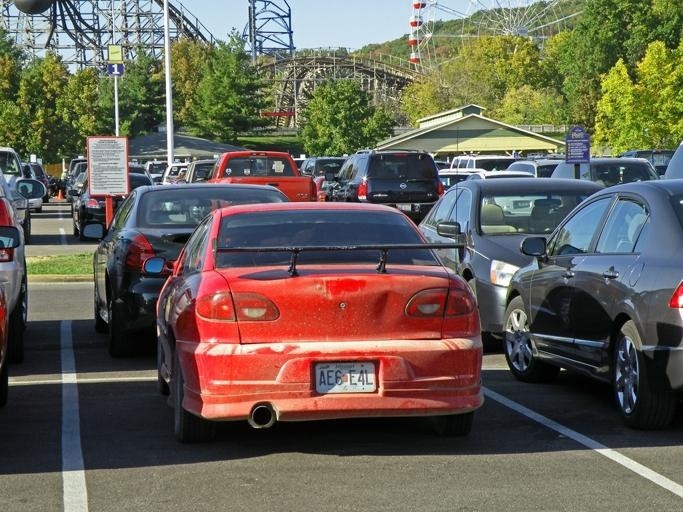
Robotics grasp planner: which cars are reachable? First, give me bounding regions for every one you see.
[417,177,608,350]
[92,182,292,359]
[501,179,682,431]
[0,167,47,368]
[140,202,485,442]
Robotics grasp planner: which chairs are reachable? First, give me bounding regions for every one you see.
[480,203,518,236]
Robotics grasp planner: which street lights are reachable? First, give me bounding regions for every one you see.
[0,224,23,406]
